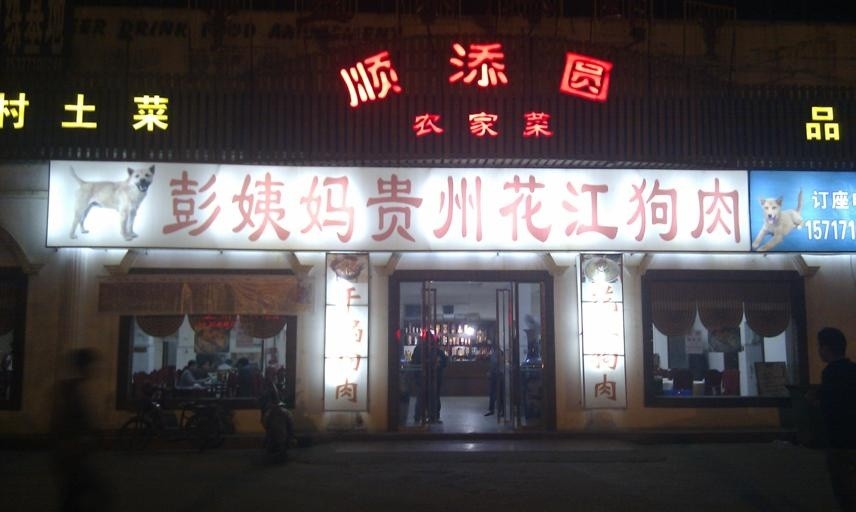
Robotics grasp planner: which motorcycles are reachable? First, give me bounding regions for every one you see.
[188,375,299,461]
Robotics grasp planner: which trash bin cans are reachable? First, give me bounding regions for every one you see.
[786,383,826,448]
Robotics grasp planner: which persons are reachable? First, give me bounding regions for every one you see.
[485,343,505,418]
[176,346,281,423]
[412,335,447,425]
[802,327,856,511]
[41,342,108,505]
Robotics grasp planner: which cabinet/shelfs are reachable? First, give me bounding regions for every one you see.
[401,330,493,362]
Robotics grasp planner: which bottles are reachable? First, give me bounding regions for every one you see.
[403,321,419,334]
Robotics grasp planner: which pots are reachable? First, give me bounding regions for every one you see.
[585,257,619,283]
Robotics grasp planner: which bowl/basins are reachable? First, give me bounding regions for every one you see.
[330,256,365,281]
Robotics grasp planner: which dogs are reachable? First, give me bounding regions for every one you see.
[68,164,155,241]
[751,191,804,252]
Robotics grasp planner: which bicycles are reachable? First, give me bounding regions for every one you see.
[120,386,224,452]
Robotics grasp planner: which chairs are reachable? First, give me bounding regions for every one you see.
[651,367,743,396]
[133,363,279,402]
